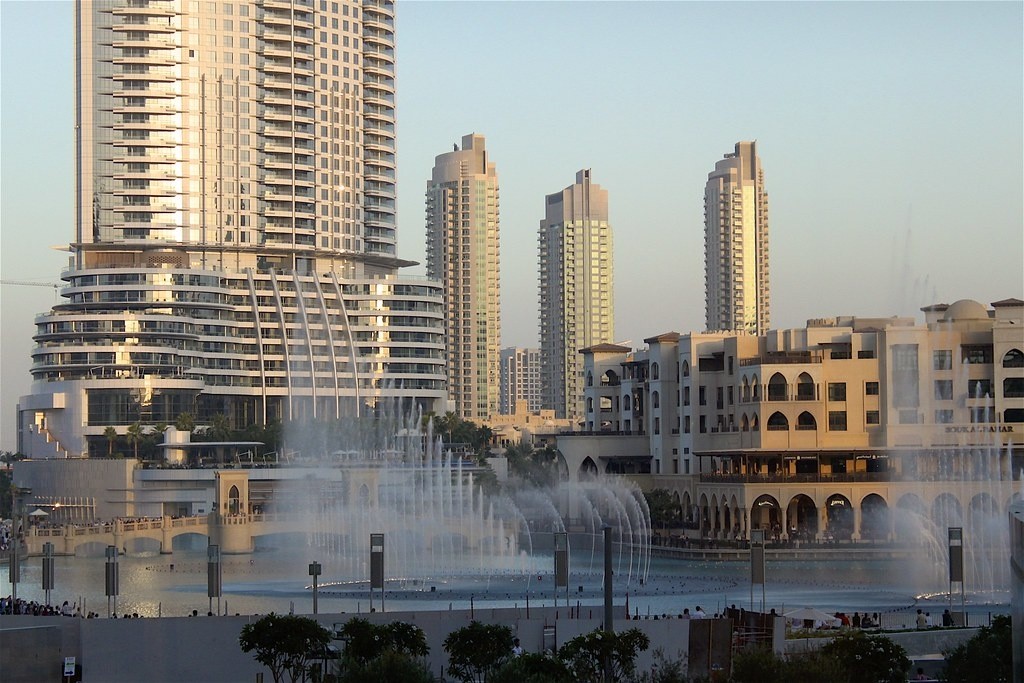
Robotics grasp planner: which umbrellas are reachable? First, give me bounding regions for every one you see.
[29,509,49,515]
[783,607,837,648]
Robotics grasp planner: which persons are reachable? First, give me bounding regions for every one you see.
[774,523,781,539]
[0,516,177,550]
[149,463,192,469]
[916,609,931,627]
[831,612,850,629]
[632,613,667,620]
[942,610,955,626]
[513,638,523,654]
[0,597,139,618]
[853,612,879,628]
[770,608,776,614]
[678,606,723,620]
